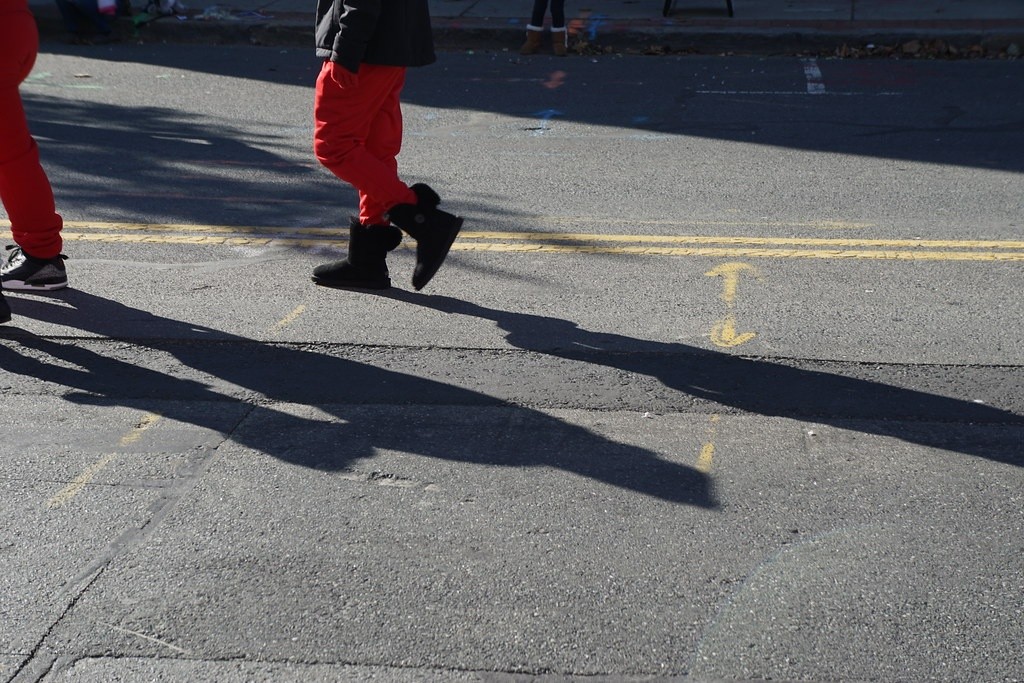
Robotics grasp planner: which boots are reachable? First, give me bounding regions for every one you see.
[309,215,402,293]
[520,24,543,53]
[550,26,569,55]
[384,183,466,290]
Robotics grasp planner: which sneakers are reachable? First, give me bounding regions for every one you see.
[0,248,68,289]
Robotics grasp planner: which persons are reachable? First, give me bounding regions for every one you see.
[311,1,464,294]
[1,0,68,326]
[520,0,569,56]
